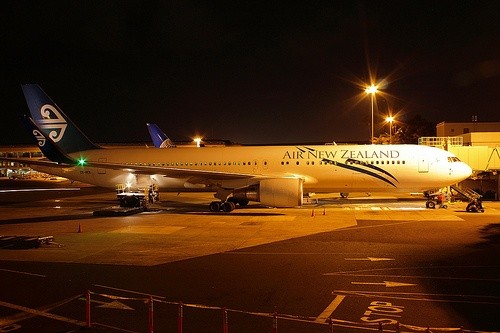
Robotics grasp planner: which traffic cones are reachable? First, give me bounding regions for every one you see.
[323,209,327,215]
[312,209,315,217]
[77,223,84,234]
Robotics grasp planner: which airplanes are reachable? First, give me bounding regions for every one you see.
[0,81,472,213]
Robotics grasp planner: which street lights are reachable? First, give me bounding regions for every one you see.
[365,84,377,143]
[385,116,393,145]
